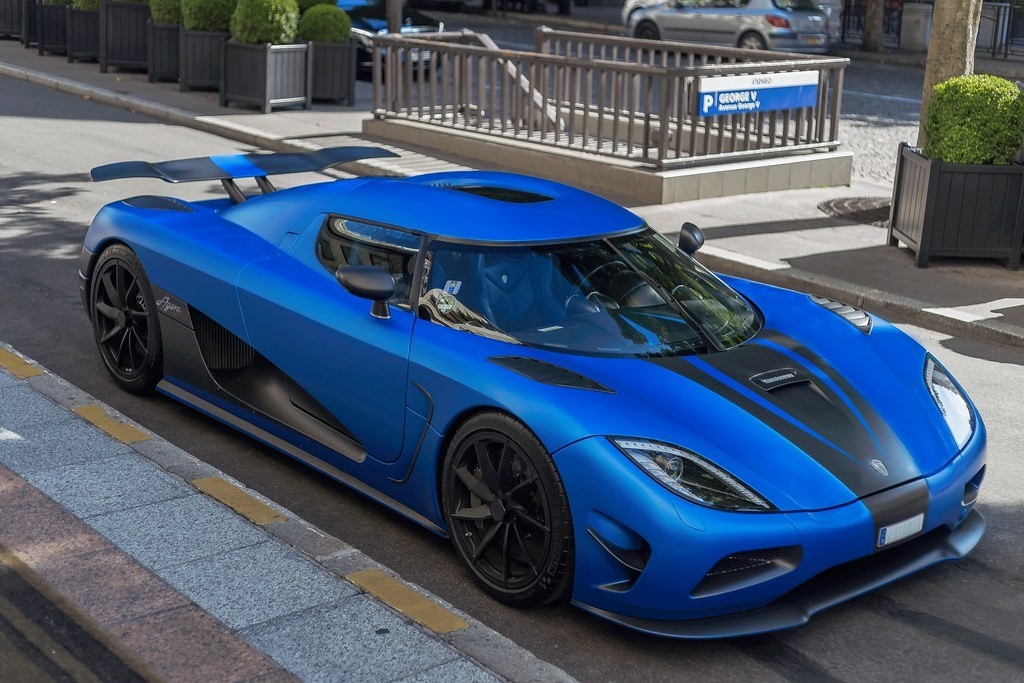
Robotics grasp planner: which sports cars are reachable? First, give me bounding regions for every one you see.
[74,146,988,641]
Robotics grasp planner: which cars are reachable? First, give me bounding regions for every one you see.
[334,1,445,80]
[629,1,831,62]
[623,0,845,48]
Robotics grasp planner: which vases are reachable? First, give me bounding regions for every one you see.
[20,0,36,47]
[0,0,20,39]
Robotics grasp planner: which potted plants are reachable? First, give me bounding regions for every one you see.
[179,0,237,93]
[886,73,1024,272]
[36,0,66,56]
[146,0,181,83]
[294,3,357,108]
[99,0,151,72]
[219,0,314,113]
[66,0,99,62]
